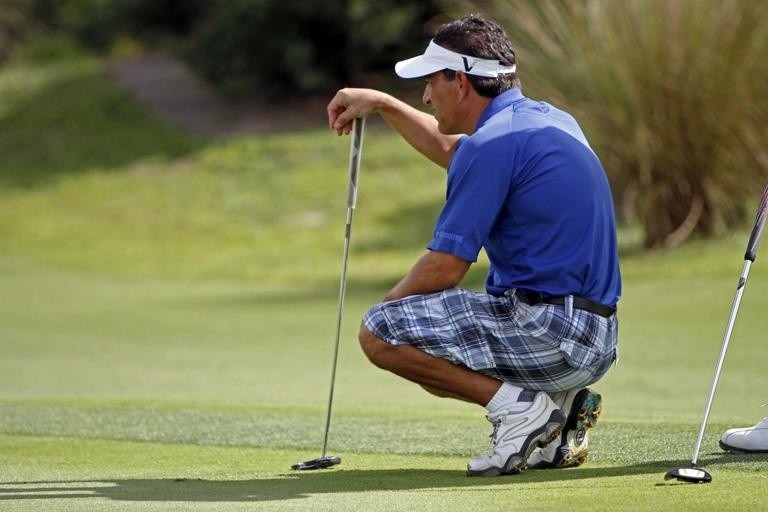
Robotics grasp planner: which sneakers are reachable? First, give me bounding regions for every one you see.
[466,388,603,476]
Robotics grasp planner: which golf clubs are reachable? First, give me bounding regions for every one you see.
[291,116,365,470]
[664,186,768,483]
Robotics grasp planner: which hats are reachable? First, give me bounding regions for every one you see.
[394,38,517,79]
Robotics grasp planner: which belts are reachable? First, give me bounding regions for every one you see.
[525,295,616,318]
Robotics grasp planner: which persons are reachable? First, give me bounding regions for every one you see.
[327,13,624,476]
